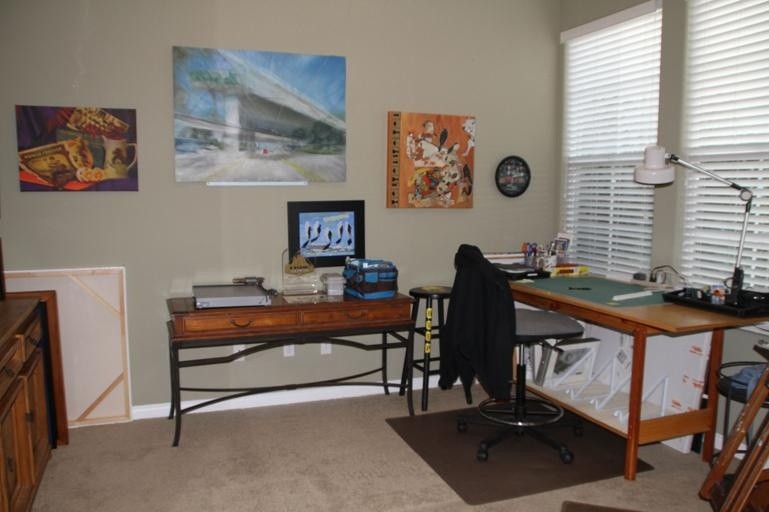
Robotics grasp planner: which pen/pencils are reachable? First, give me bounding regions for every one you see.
[568,287,592,290]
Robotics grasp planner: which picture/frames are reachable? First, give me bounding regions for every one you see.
[287,199,365,268]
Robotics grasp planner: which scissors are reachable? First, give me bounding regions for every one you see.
[521,241,537,267]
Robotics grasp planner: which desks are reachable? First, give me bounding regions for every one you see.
[495,274,768,481]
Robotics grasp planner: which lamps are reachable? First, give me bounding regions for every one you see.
[633,147,753,304]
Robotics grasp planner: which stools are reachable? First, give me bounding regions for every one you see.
[399,286,476,411]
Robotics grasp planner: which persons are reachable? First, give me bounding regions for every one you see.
[45,156,66,176]
[407,119,449,200]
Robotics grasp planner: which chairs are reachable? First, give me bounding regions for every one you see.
[456,244,585,464]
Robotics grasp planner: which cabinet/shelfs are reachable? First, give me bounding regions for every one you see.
[166,288,415,448]
[0,298,53,511]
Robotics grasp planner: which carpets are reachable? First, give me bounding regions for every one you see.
[385,399,654,511]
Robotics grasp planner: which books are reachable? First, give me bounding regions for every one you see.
[493,263,536,274]
